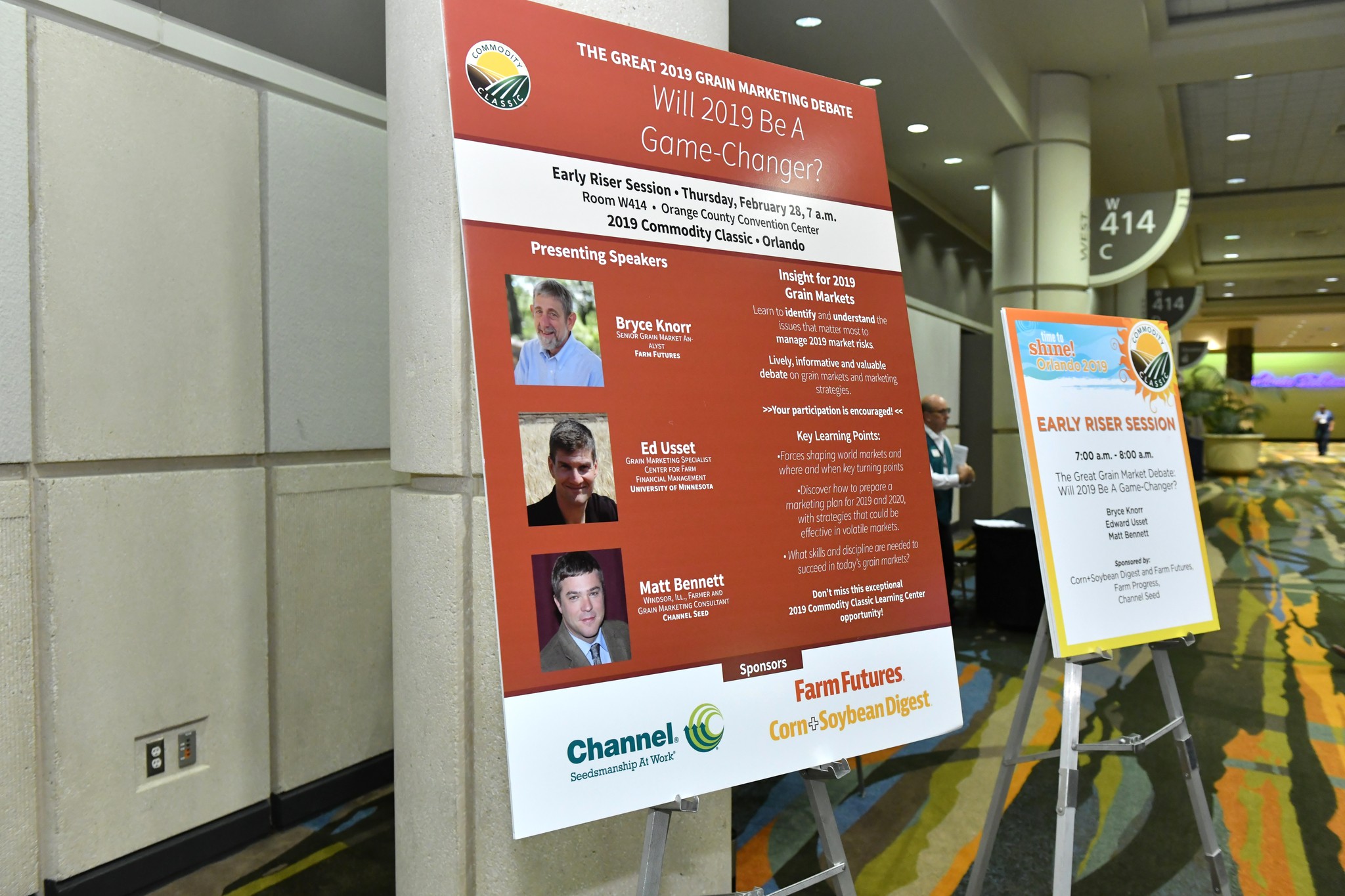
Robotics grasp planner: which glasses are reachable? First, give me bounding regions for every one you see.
[931,406,951,416]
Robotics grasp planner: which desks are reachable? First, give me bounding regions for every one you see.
[970,508,1046,631]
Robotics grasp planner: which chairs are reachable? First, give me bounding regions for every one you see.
[956,548,977,601]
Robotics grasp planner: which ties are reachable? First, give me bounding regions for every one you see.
[590,643,602,665]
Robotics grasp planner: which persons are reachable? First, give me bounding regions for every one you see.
[514,280,605,387]
[1313,404,1335,456]
[539,551,632,673]
[921,394,976,621]
[527,419,618,527]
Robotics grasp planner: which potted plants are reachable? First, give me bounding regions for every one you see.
[1201,399,1270,477]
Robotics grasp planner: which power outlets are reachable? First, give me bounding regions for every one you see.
[146,738,165,779]
